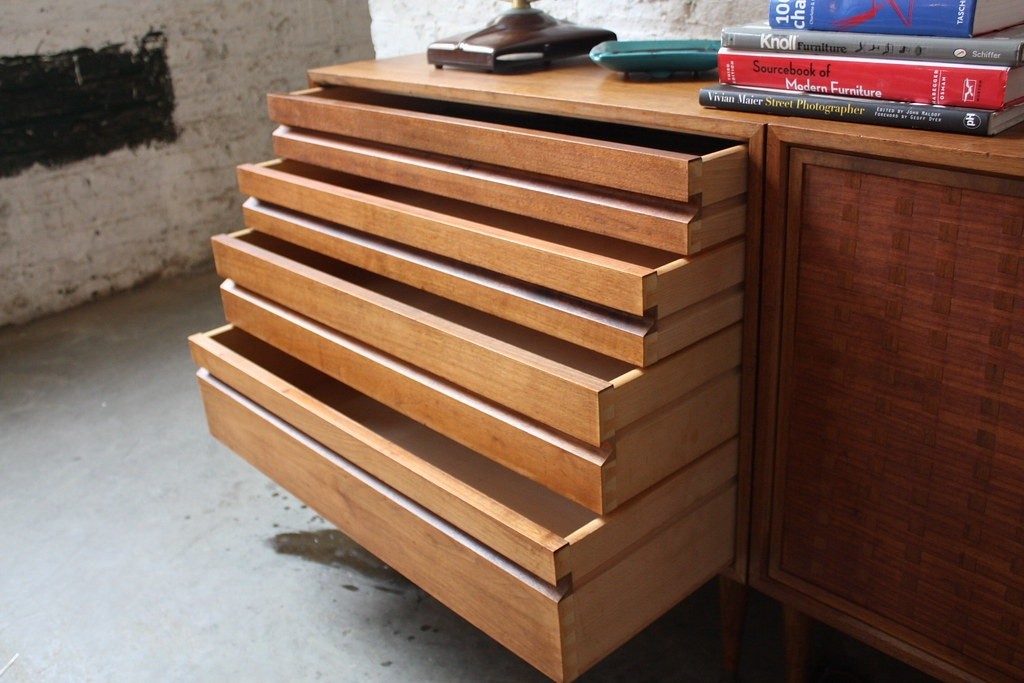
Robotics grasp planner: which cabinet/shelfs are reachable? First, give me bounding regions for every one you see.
[188,41,1024,683]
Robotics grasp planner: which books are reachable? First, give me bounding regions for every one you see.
[699,0,1024,136]
